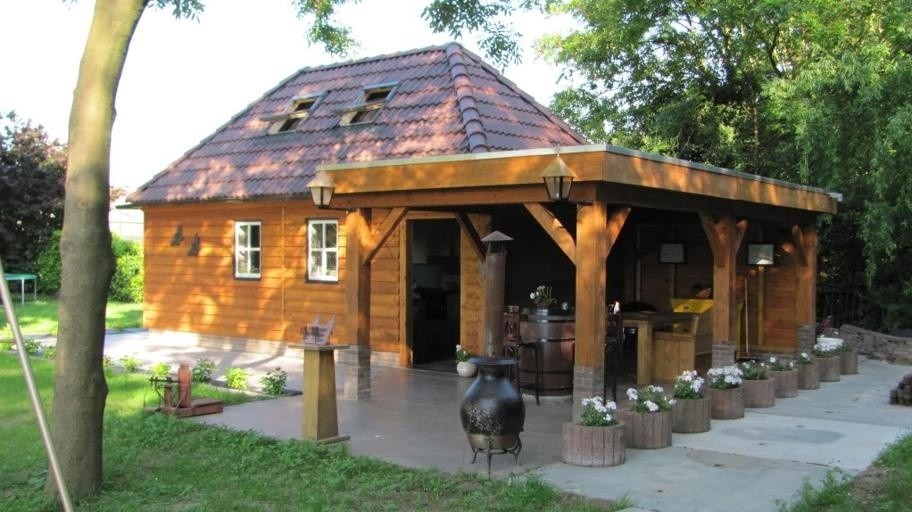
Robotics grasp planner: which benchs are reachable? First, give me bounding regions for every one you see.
[652,310,713,385]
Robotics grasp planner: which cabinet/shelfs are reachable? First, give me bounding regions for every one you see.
[667,295,746,361]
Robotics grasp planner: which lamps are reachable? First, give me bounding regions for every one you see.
[537,142,592,208]
[301,159,354,215]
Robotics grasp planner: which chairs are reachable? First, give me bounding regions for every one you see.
[502,312,544,406]
[572,311,625,404]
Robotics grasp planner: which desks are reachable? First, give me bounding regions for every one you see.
[606,310,700,387]
[516,311,577,398]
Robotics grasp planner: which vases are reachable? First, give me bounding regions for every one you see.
[458,355,528,452]
[536,302,549,316]
[455,361,478,378]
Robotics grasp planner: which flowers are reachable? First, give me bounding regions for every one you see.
[454,343,473,363]
[560,341,859,470]
[528,283,551,304]
